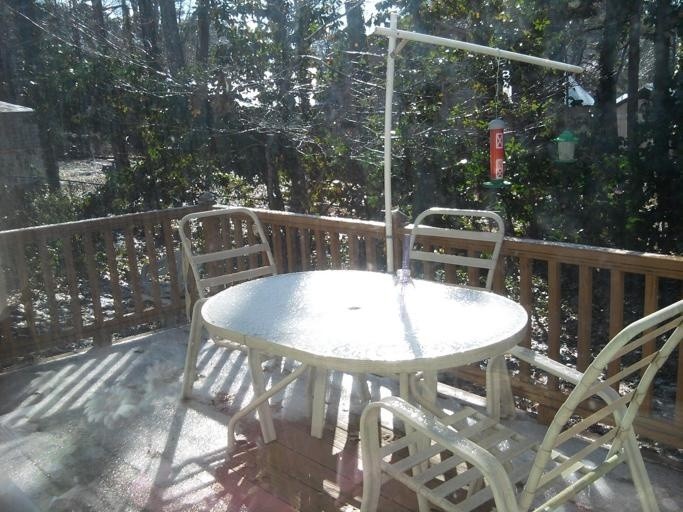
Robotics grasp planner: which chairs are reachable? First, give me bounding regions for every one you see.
[398,205,516,417]
[361,303,681,510]
[180,207,280,444]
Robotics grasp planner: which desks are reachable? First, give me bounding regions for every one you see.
[200,270,529,510]
